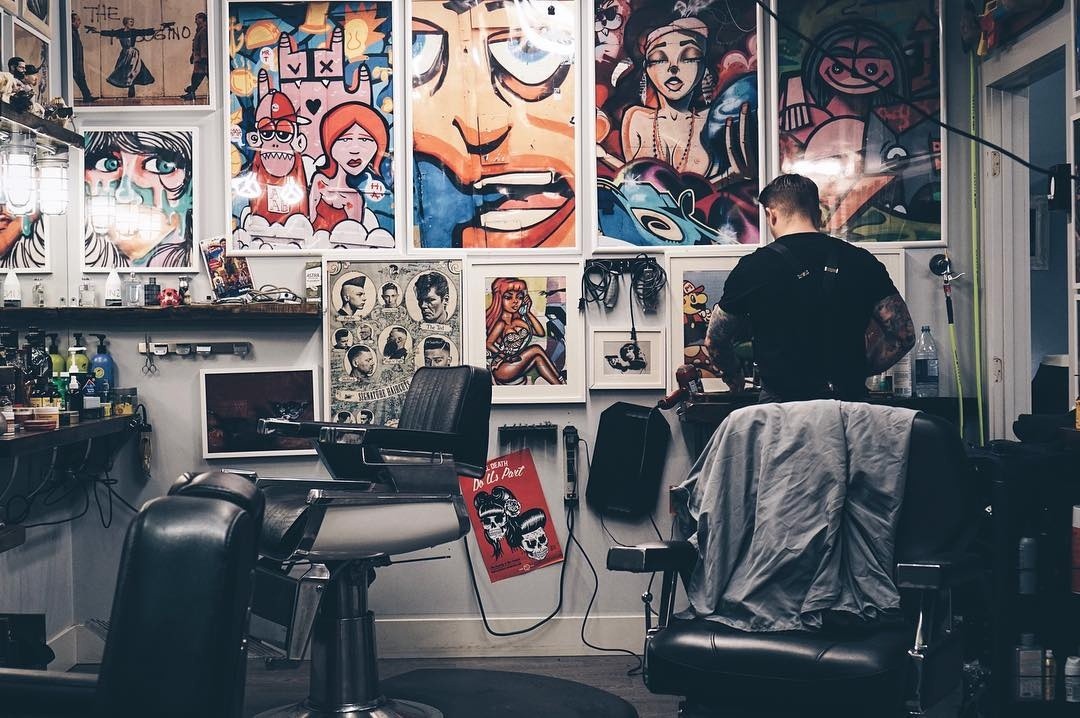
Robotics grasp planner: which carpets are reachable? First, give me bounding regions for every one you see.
[379,668,638,718]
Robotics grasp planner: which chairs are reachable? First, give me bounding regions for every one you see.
[606,407,976,718]
[247,365,493,718]
[0,469,262,717]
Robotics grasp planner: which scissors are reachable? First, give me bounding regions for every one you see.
[142,334,156,374]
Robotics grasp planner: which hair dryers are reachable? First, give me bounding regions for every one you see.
[657,363,705,409]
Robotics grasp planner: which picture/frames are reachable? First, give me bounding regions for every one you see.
[12,15,52,105]
[468,259,587,404]
[403,0,581,255]
[589,326,666,389]
[200,365,319,458]
[770,0,947,250]
[321,255,469,427]
[587,0,766,253]
[666,248,907,396]
[65,0,217,111]
[0,202,53,273]
[78,127,200,273]
[222,0,400,256]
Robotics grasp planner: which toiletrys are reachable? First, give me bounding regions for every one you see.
[90,334,115,389]
[104,263,122,306]
[144,278,161,304]
[0,325,100,424]
[121,272,145,306]
[64,333,90,374]
[45,332,66,374]
[31,277,44,308]
[78,275,95,307]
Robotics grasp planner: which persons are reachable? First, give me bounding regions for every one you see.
[337,276,367,316]
[0,57,39,114]
[179,12,208,101]
[424,338,452,368]
[212,238,249,294]
[361,411,372,425]
[84,18,176,98]
[384,327,408,359]
[72,13,99,102]
[333,329,351,350]
[413,272,449,322]
[381,283,399,308]
[337,410,353,424]
[360,325,372,341]
[703,173,916,404]
[348,346,374,378]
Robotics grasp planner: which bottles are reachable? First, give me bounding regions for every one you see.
[32,278,45,308]
[123,271,143,306]
[892,350,912,398]
[914,326,939,397]
[28,372,83,423]
[1013,633,1056,676]
[79,276,95,307]
[1015,676,1056,704]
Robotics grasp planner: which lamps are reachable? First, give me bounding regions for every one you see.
[35,144,73,214]
[0,129,38,215]
